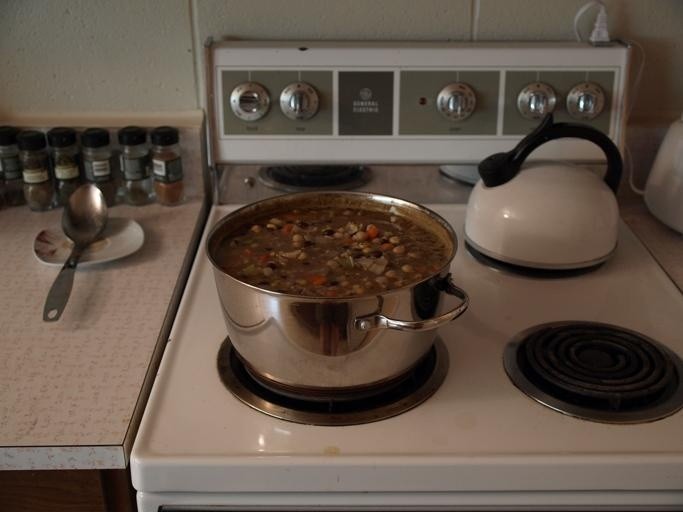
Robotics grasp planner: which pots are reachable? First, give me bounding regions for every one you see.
[202,187,471,396]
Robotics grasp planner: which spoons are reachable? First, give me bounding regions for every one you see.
[40,183,109,322]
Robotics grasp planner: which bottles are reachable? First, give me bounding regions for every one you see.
[148,124,185,206]
[79,125,118,208]
[116,125,151,207]
[0,124,24,206]
[46,125,83,208]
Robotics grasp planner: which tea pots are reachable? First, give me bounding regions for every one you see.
[460,110,625,279]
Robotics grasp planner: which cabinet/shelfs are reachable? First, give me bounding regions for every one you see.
[0,464,136,512]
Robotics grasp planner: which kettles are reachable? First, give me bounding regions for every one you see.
[16,129,55,213]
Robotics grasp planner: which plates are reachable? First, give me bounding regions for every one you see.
[30,213,146,271]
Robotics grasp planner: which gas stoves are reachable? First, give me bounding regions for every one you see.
[129,201,682,495]
